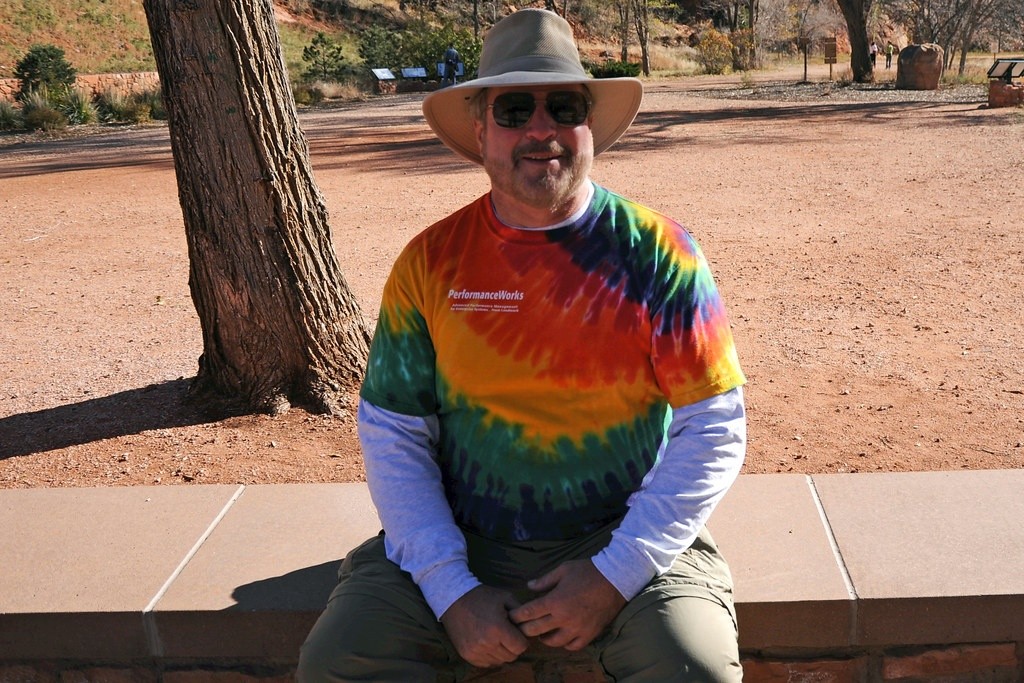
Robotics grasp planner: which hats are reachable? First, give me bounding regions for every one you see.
[422,7,645,163]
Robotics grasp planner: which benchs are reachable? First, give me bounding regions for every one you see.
[0,467,1024,682]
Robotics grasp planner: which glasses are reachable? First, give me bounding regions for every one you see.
[479,89,594,130]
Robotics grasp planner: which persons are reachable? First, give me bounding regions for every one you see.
[295,8,748,681]
[869,42,878,68]
[441,43,459,87]
[885,41,894,68]
